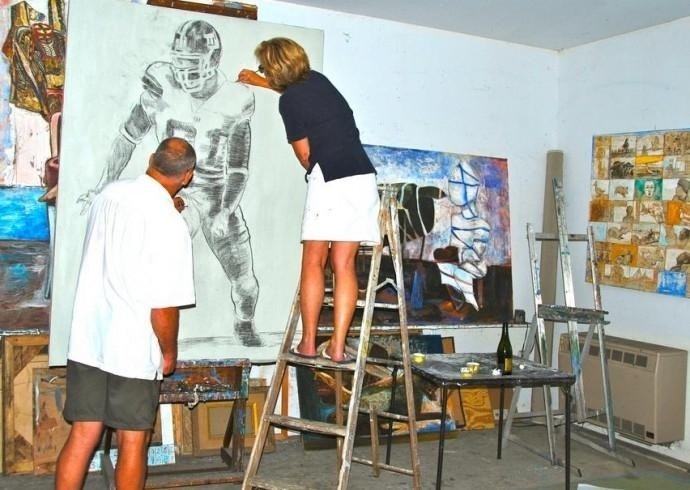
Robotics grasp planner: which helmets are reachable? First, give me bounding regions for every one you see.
[171,19,220,78]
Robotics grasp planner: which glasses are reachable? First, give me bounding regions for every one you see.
[256,65,266,73]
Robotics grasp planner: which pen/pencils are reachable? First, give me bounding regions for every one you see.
[235,65,264,83]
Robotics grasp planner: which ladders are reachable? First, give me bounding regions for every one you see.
[240,187,423,490]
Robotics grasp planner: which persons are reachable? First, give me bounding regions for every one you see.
[673,178,690,201]
[237,37,380,364]
[77,20,260,350]
[55,137,195,490]
[639,180,659,200]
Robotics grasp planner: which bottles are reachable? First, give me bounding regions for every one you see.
[496,322,513,375]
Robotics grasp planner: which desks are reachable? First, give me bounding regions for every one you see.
[383,351,577,490]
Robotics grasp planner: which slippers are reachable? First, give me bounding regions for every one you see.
[288,344,321,359]
[321,347,352,365]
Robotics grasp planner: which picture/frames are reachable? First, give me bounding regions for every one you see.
[172,379,277,458]
[0,329,176,475]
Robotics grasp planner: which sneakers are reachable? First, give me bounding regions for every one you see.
[233,316,262,347]
